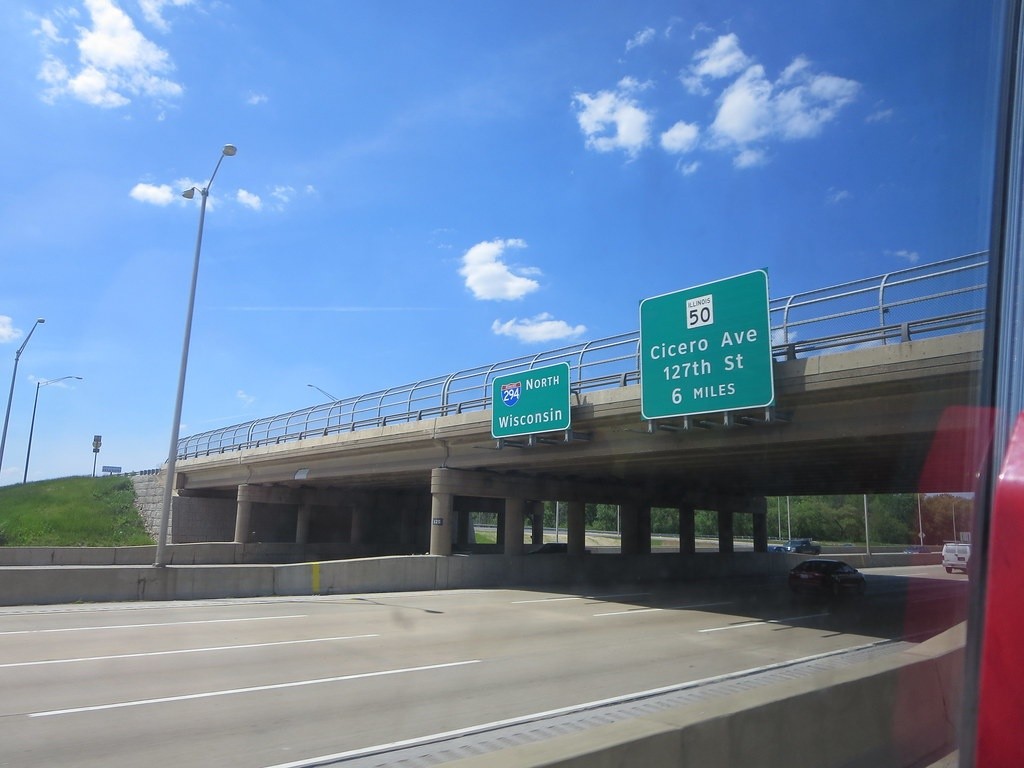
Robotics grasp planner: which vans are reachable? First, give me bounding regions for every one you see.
[942,543,971,573]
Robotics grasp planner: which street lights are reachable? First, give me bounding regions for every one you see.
[156,144,237,568]
[307,384,338,401]
[0,318,45,473]
[23,376,83,485]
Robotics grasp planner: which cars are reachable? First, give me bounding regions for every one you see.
[904,545,930,553]
[958,0,1023,768]
[768,544,788,554]
[785,539,821,554]
[790,559,867,600]
[528,542,591,554]
